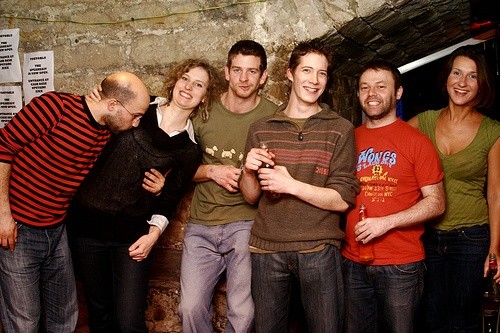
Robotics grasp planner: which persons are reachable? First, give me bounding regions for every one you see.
[66,57,228,333]
[0,71,150,333]
[239,39,361,333]
[405,46,500,333]
[178,41,278,333]
[341,61,445,333]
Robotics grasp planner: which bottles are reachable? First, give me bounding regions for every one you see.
[483,253,500,333]
[357,205,375,262]
[258,140,283,201]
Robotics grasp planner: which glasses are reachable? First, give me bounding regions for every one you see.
[116,100,143,122]
